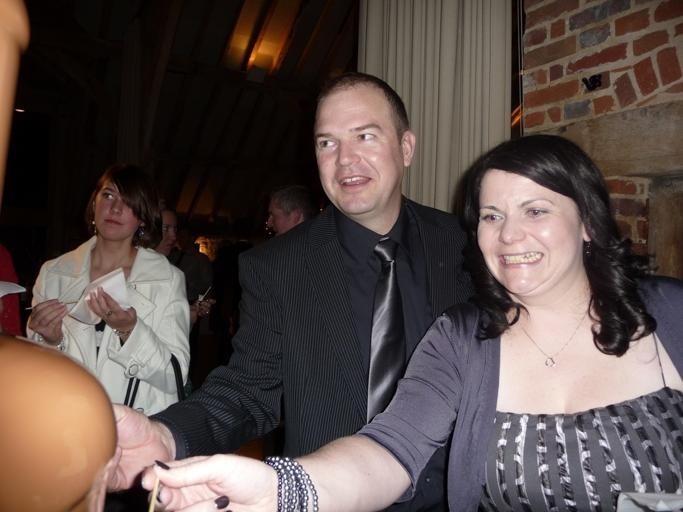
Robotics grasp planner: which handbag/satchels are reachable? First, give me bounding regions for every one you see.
[102,352,185,512]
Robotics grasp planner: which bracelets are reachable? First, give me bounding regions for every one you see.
[116,328,133,336]
[263,456,319,512]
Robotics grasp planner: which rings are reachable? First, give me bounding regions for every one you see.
[106,311,112,315]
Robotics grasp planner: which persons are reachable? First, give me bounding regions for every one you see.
[145,198,252,366]
[266,185,315,236]
[144,133,683,512]
[28,161,190,512]
[102,72,472,512]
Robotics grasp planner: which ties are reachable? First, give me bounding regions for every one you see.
[365,234,409,431]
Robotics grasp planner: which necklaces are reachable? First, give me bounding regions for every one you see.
[522,309,589,369]
